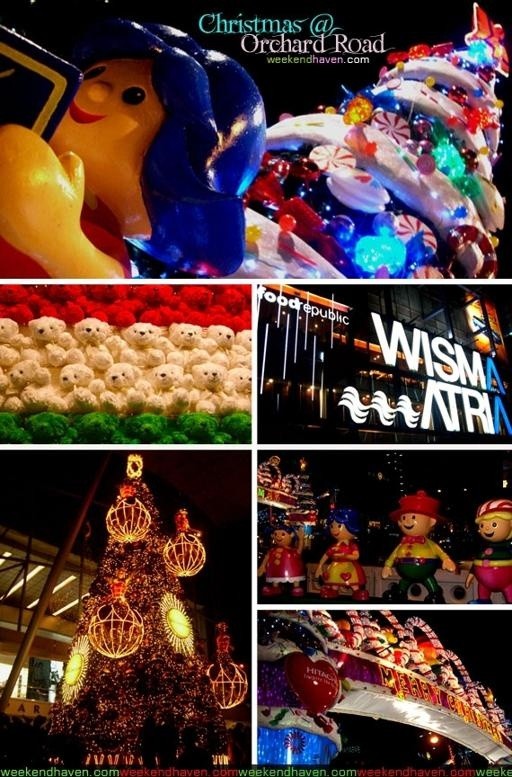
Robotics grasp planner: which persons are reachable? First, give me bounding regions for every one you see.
[460,498,512,604]
